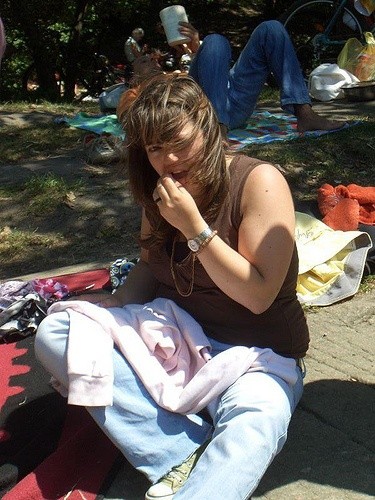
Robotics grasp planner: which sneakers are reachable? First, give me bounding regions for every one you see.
[145,432,208,500]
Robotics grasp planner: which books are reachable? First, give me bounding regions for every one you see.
[160,5,191,46]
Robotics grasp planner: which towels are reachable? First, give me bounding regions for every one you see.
[317,182,375,231]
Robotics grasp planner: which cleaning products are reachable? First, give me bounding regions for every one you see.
[356,54,372,81]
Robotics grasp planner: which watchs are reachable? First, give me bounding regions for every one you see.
[187,227,210,252]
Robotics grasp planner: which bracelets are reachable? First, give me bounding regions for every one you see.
[193,229,218,255]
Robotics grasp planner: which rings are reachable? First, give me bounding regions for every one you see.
[154,197,161,203]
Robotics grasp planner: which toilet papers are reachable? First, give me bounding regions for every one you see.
[160,4,191,47]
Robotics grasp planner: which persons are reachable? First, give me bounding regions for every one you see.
[124,22,148,63]
[117,20,347,147]
[35,75,310,500]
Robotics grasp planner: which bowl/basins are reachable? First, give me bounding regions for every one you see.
[340,80,375,102]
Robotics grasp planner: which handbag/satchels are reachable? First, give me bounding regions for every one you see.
[0,281,50,342]
